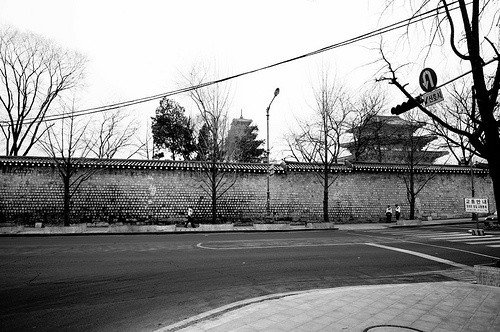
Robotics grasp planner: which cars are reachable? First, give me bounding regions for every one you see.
[484,210,500,231]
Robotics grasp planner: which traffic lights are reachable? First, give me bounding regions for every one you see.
[391,98,422,115]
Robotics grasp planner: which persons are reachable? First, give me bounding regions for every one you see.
[395,203,400,221]
[184,206,196,228]
[385,205,392,223]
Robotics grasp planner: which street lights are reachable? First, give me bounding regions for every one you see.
[265,88,280,224]
